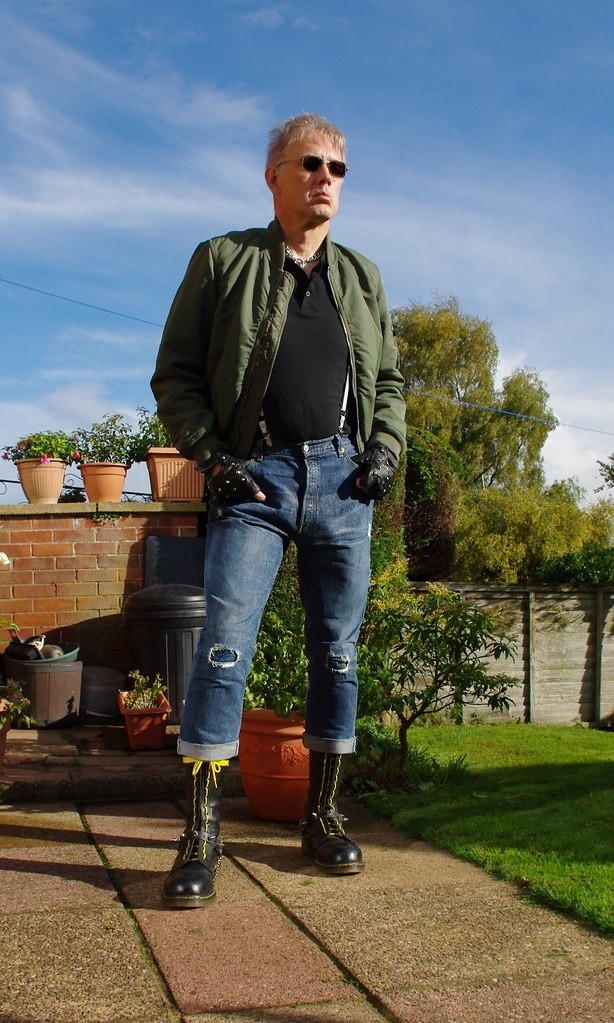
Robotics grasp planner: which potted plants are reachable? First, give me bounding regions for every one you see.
[241,513,414,822]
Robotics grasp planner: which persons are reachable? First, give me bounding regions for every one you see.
[150,117,406,908]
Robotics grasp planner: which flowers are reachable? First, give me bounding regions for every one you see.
[117,670,169,710]
[0,407,176,467]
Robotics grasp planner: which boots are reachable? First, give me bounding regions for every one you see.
[303,749,365,874]
[160,757,230,908]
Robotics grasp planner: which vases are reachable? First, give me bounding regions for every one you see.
[76,463,131,502]
[144,447,203,503]
[119,689,171,750]
[12,458,69,505]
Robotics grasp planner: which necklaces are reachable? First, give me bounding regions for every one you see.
[285,244,324,269]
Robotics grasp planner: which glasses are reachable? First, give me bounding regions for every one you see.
[276,154,350,178]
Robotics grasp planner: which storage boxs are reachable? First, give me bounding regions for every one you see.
[5,642,82,730]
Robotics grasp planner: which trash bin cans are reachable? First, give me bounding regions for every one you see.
[121,582,210,740]
